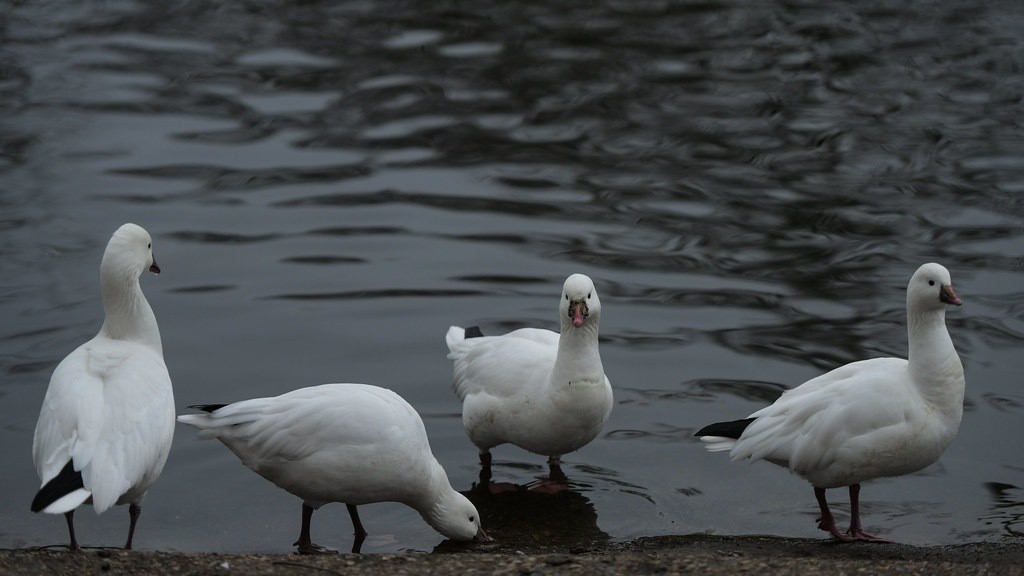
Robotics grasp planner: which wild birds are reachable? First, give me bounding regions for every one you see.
[32,221,176,553]
[693,261,965,542]
[177,381,494,555]
[444,272,615,466]
